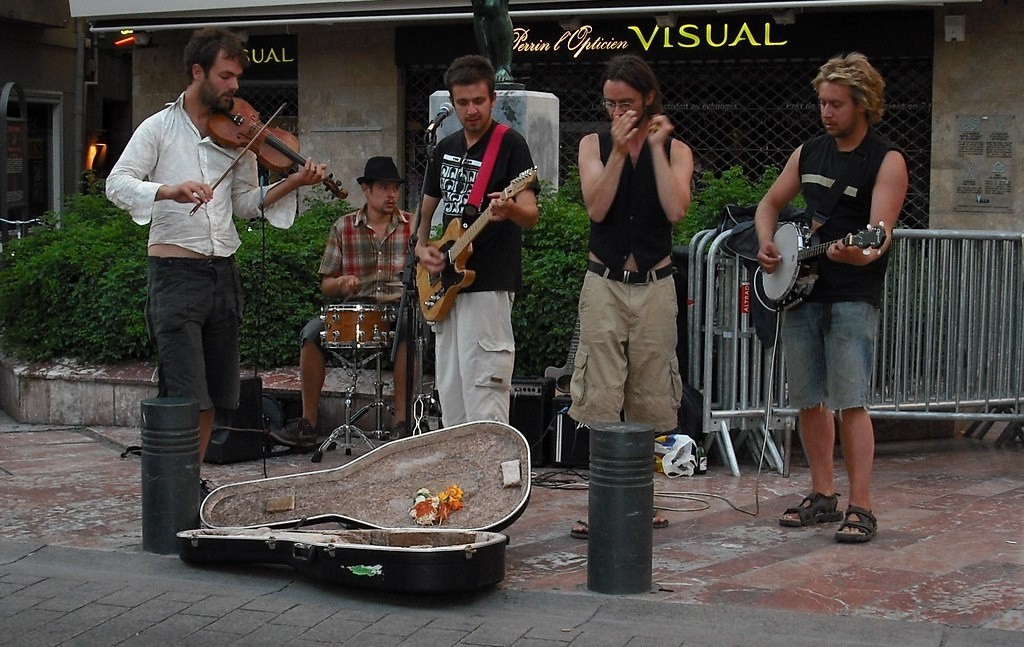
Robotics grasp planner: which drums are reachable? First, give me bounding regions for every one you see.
[319,303,394,351]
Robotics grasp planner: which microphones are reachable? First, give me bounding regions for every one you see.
[426,102,454,135]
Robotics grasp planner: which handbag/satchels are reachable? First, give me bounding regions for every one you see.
[653,434,698,478]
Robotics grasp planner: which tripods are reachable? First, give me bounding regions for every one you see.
[310,344,394,465]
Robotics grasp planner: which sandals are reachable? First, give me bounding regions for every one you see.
[653,510,669,528]
[834,507,877,542]
[570,520,588,538]
[779,493,843,526]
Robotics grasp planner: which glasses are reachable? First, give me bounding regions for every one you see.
[602,97,642,111]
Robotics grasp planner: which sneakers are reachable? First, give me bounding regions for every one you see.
[269,418,317,447]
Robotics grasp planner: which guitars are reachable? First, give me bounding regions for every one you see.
[414,165,540,326]
[761,218,888,310]
[207,95,349,200]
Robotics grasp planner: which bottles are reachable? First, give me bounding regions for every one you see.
[695,441,707,475]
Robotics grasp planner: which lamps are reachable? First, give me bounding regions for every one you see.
[655,12,680,28]
[134,31,151,47]
[772,7,795,24]
[559,15,581,31]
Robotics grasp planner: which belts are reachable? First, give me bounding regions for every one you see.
[586,260,672,284]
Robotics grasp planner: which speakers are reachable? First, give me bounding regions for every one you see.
[553,393,624,469]
[203,376,266,465]
[506,377,558,467]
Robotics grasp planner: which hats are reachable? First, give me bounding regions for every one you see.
[357,157,409,185]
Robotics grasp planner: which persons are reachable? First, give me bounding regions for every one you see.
[470,0,526,90]
[754,51,908,541]
[106,27,327,504]
[566,53,694,540]
[269,156,418,442]
[414,54,541,428]
